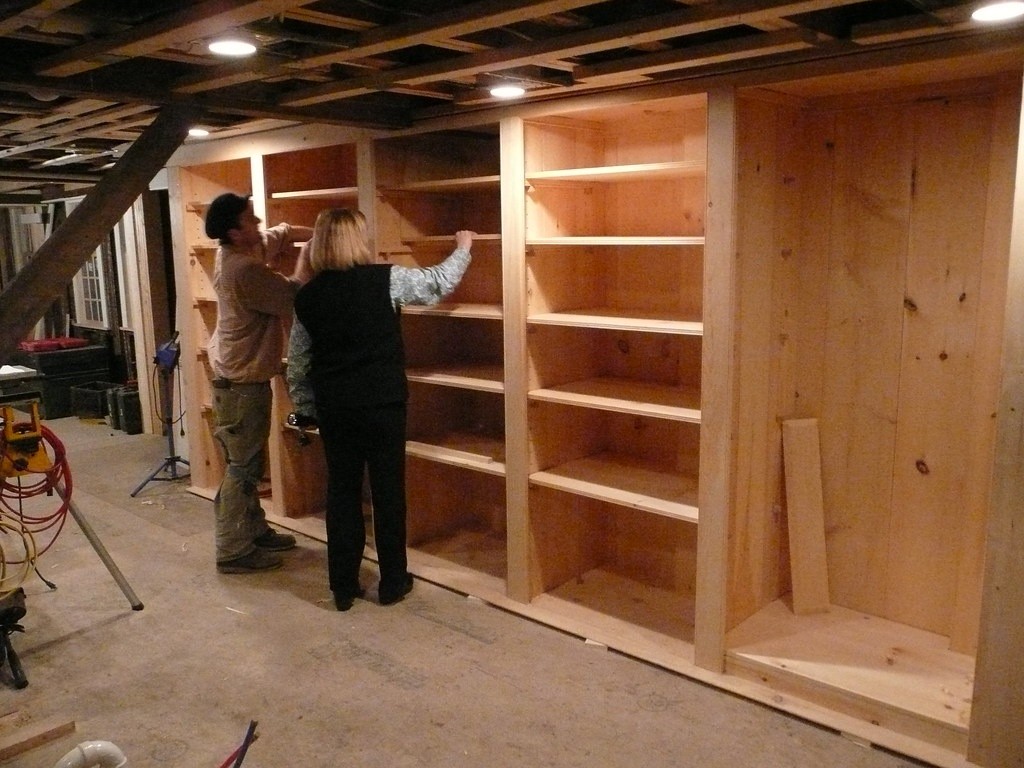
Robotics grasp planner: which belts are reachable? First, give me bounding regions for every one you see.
[211,379,224,389]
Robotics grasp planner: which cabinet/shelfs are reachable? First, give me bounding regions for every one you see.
[167,24,1023,767]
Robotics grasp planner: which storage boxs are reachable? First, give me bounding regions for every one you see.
[71,381,124,420]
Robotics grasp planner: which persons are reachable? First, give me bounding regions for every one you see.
[284,207,476,611]
[204,193,316,574]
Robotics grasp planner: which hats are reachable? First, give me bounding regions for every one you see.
[205,192,252,239]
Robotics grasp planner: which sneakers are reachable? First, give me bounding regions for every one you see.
[216,550,284,573]
[252,527,297,551]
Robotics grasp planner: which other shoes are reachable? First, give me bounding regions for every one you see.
[378,571,414,605]
[334,575,360,611]
